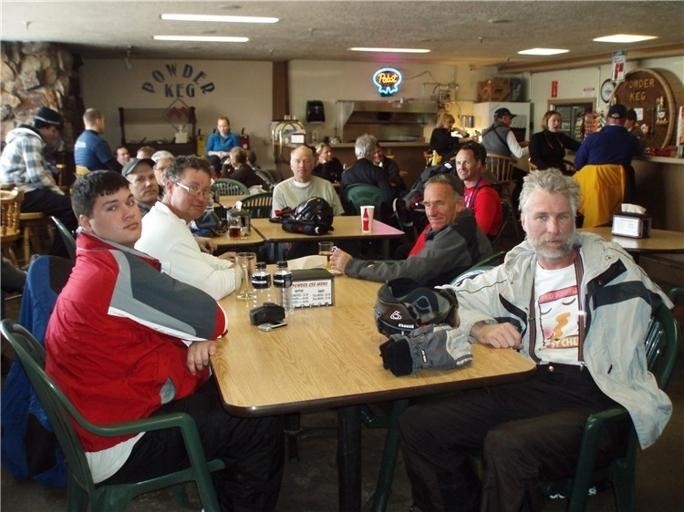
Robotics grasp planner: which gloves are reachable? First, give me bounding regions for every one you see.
[283,220,327,235]
[379,324,473,376]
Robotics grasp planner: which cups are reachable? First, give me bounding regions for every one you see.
[317,242,334,269]
[358,206,376,232]
[234,251,256,302]
[228,227,240,239]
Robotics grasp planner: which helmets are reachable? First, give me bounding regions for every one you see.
[374,278,459,335]
[291,197,333,225]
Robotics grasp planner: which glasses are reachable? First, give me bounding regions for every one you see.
[176,182,211,195]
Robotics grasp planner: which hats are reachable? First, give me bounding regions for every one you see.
[608,104,625,118]
[34,107,60,125]
[494,109,516,119]
[122,158,155,175]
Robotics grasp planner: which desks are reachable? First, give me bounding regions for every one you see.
[208,253,539,419]
[575,225,683,253]
[199,215,406,247]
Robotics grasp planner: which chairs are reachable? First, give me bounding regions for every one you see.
[372,285,680,511]
[71,152,625,217]
[1,159,67,270]
[2,318,229,511]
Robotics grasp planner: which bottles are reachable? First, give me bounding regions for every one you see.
[272,259,293,315]
[212,187,219,204]
[462,114,473,128]
[251,261,270,307]
[362,208,369,231]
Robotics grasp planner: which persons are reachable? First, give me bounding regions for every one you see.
[573,105,647,204]
[479,107,530,159]
[271,144,344,217]
[134,154,247,299]
[454,141,502,236]
[429,113,472,158]
[340,134,404,205]
[330,172,492,291]
[40,168,286,511]
[527,110,582,175]
[204,116,268,190]
[311,142,347,183]
[1,106,176,233]
[392,168,675,511]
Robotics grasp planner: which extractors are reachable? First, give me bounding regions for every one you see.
[340,100,439,125]
